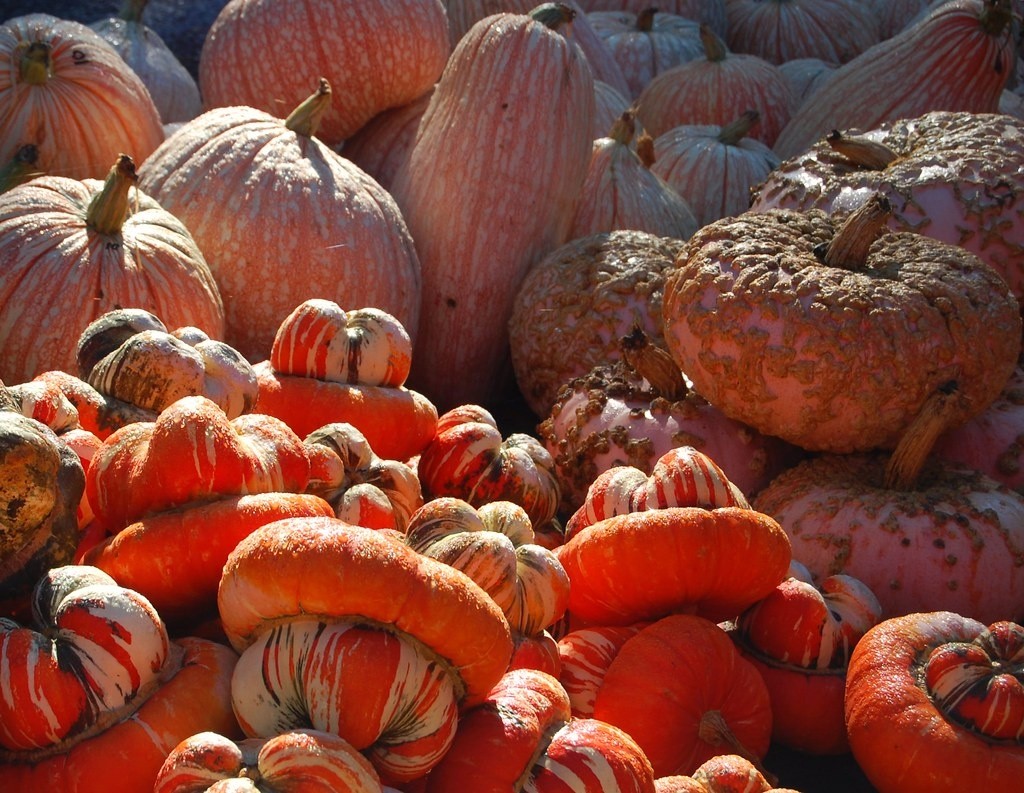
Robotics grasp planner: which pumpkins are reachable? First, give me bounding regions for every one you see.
[1,0,1024,792]
